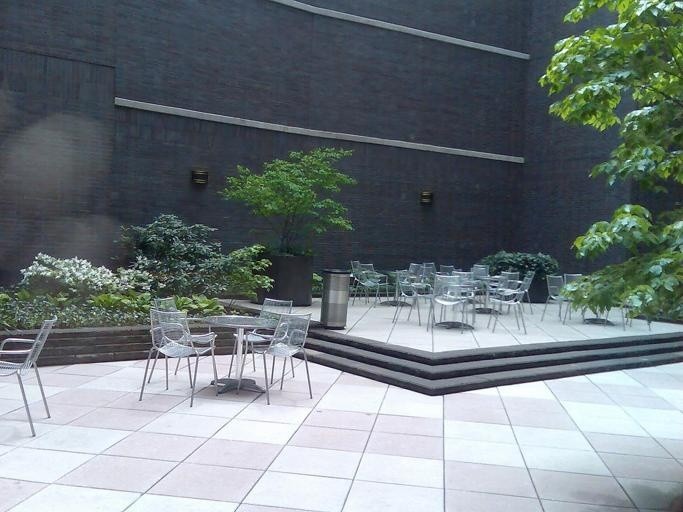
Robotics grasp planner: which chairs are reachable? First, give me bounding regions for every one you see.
[138,297,315,407]
[0,314,58,437]
[349,259,586,335]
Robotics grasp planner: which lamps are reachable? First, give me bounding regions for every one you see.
[419,192,433,206]
[191,170,208,185]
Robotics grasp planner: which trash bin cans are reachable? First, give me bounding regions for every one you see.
[321,269,351,330]
